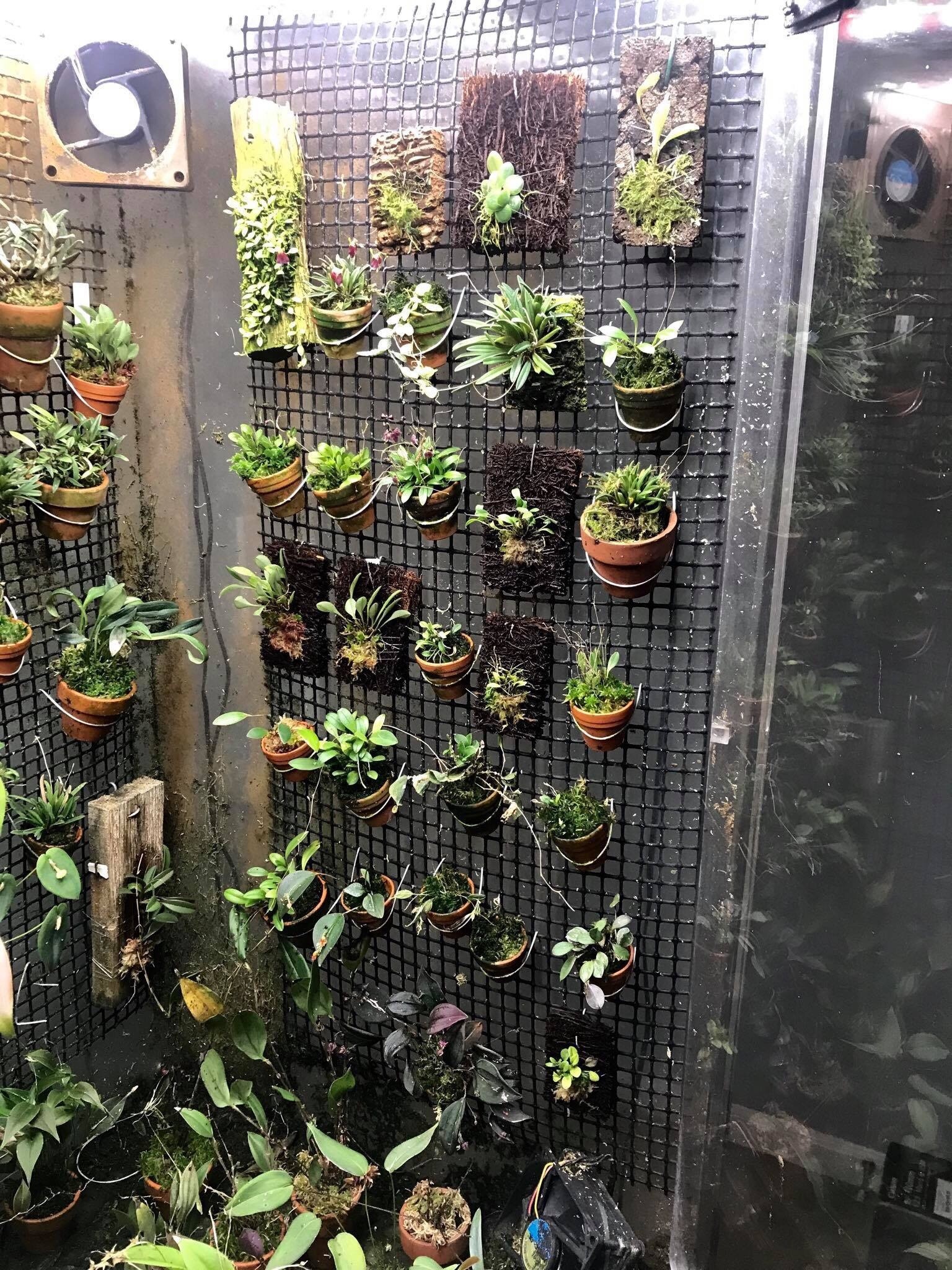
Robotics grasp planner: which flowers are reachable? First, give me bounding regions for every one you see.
[307,240,391,304]
[376,421,467,508]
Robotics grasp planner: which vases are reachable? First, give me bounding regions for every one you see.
[309,296,373,359]
[396,481,460,541]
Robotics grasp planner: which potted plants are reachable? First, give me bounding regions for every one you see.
[0,206,685,1270]
